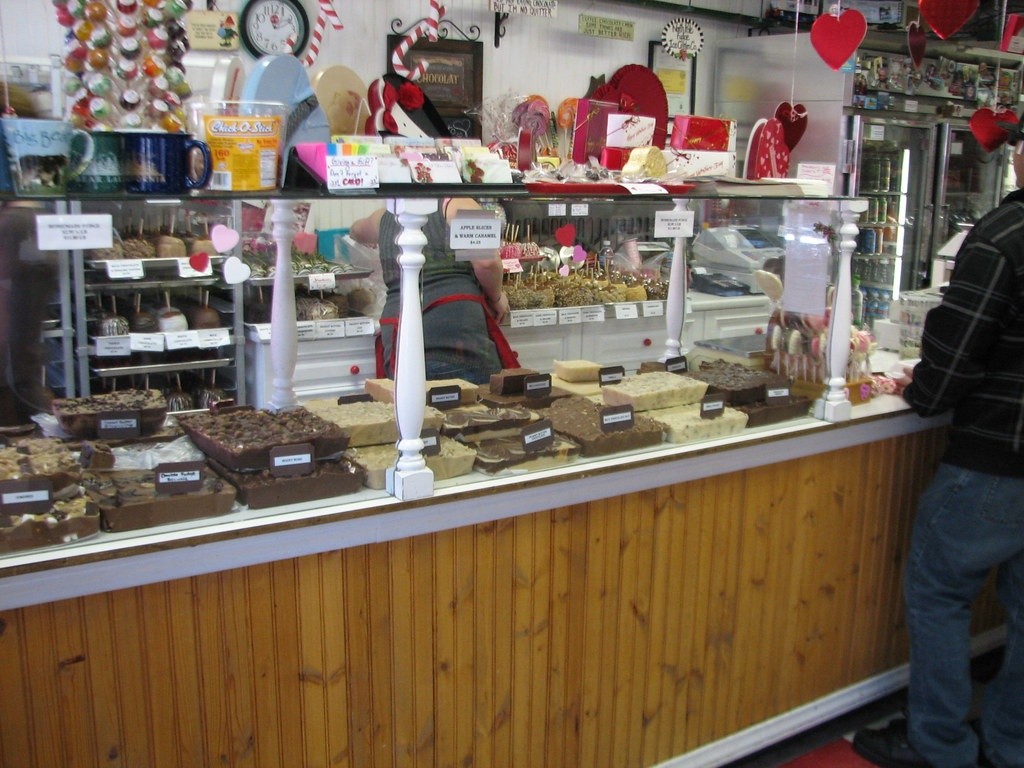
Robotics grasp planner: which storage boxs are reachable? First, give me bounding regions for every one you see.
[669,115,732,151]
[601,146,635,170]
[204,100,287,192]
[661,147,736,179]
[606,113,656,148]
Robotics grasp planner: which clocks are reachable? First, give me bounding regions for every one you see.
[239,0,310,63]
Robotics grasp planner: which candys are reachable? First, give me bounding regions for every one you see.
[52,0,193,133]
[756,254,872,381]
[511,95,579,160]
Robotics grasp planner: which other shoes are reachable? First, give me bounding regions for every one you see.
[968,719,996,768]
[853,704,934,768]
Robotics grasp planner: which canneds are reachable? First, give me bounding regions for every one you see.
[854,158,892,254]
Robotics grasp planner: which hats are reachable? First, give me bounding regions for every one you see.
[998,113,1024,134]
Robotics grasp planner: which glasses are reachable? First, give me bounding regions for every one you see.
[1008,132,1024,146]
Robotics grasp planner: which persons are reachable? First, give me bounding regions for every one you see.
[351,196,514,390]
[853,115,1024,768]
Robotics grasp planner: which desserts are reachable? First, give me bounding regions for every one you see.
[83,205,375,411]
[0,361,815,557]
[498,223,672,312]
